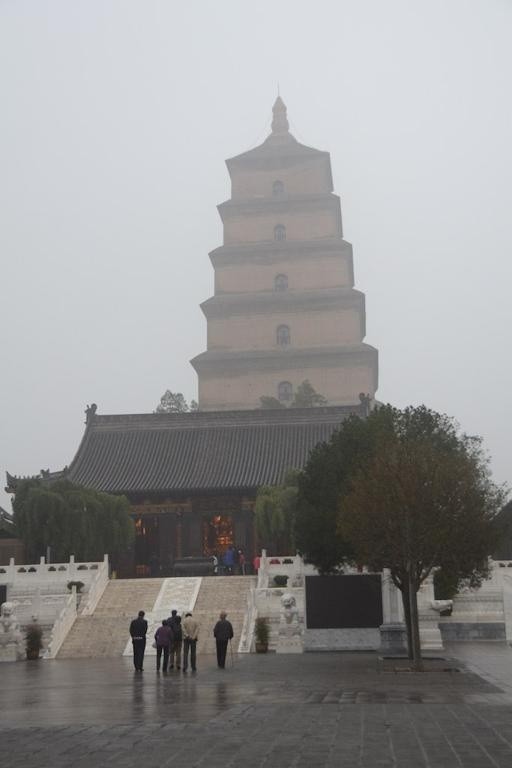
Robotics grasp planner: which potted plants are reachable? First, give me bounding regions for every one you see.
[254,618,269,652]
[25,625,41,659]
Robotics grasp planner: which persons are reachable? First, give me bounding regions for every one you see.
[208,543,261,575]
[168,616,182,670]
[179,612,200,673]
[165,609,181,625]
[129,610,148,671]
[154,619,175,673]
[213,611,234,668]
[130,669,233,725]
